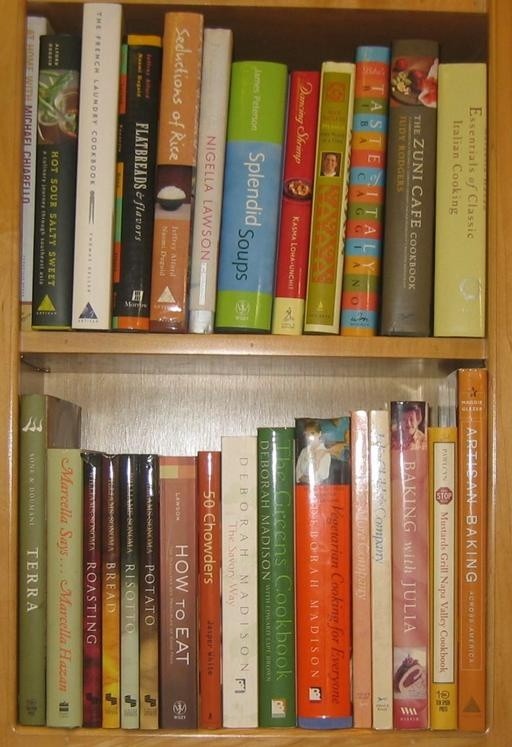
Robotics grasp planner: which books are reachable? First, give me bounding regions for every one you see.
[17,366,489,734]
[13,0,490,339]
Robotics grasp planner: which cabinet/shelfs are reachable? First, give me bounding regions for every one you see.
[1,2,512,747]
